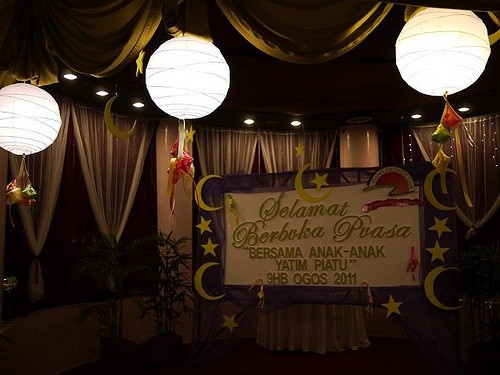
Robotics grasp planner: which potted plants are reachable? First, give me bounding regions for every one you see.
[120,230,194,371]
[449,238,500,375]
[62,230,144,375]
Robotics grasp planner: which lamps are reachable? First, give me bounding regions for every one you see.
[0,81,63,230]
[394,4,490,210]
[144,32,233,220]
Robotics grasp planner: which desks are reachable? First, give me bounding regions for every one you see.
[255,293,371,353]
